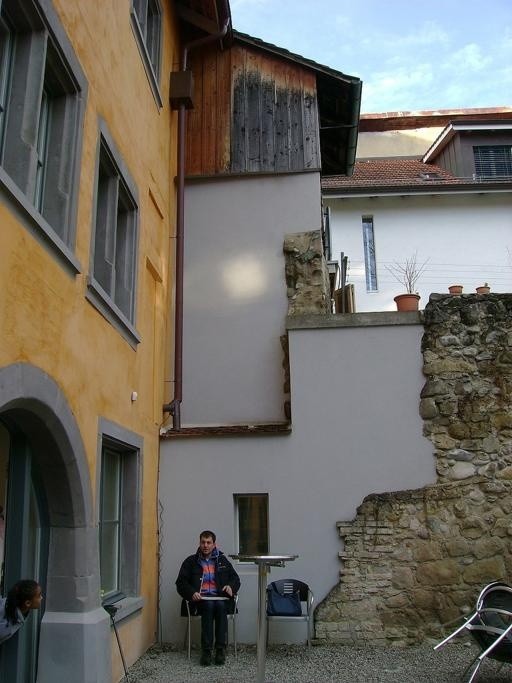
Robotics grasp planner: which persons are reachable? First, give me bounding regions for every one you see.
[175,529,241,667]
[0,578,44,643]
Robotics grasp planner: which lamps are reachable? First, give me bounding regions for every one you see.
[362,214,377,293]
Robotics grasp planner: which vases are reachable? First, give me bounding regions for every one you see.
[448,285,463,294]
[476,287,490,295]
[394,294,421,312]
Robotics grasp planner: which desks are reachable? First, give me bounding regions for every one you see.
[227,554,299,683]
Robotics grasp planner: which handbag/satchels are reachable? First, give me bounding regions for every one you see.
[268,590,302,616]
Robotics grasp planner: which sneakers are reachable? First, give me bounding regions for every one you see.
[200,649,211,666]
[215,648,225,665]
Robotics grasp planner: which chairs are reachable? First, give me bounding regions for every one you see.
[183,592,238,660]
[431,581,512,683]
[264,578,314,648]
